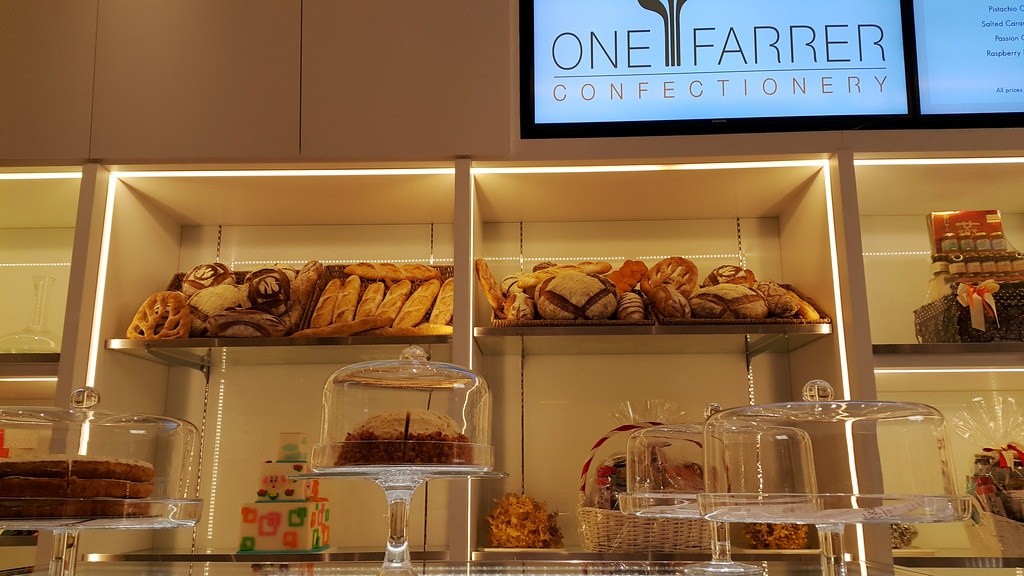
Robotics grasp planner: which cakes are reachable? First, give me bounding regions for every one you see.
[332,409,476,467]
[239,432,332,553]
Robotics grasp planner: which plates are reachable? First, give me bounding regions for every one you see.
[477,547,566,552]
[744,550,820,554]
[892,550,940,553]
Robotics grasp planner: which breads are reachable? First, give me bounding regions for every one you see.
[475,254,820,321]
[125,261,455,335]
[0,453,154,519]
[592,457,706,510]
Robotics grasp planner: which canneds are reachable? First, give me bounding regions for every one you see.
[930,230,1024,297]
[973,453,1024,481]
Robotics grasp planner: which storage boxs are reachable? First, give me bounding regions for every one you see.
[0,429,38,448]
[926,209,1004,253]
[0,448,35,458]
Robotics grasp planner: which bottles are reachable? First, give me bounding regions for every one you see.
[0,275,61,353]
[976,476,1007,518]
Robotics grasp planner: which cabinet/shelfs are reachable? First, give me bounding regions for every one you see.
[0,148,1024,576]
